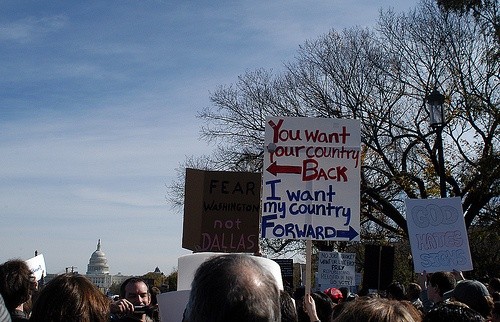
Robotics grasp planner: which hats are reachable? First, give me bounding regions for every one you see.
[443,280,490,303]
[324,288,343,298]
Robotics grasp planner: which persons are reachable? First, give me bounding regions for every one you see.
[0,258,177,322]
[181,254,281,322]
[281,268,500,322]
